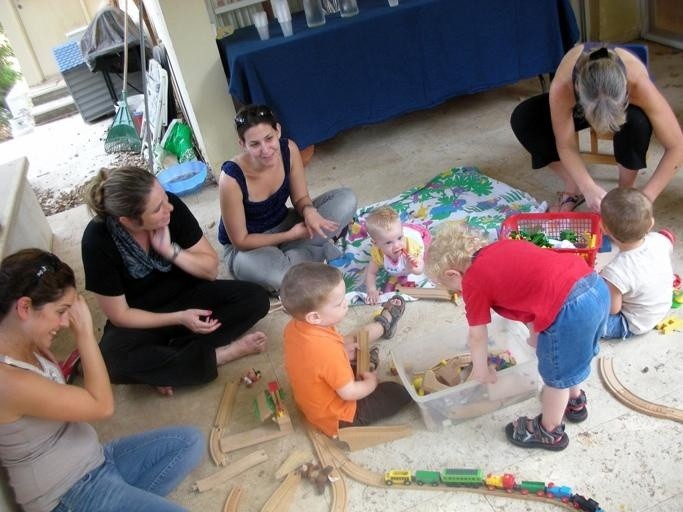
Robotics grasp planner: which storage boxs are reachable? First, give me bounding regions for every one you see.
[389,310,539,433]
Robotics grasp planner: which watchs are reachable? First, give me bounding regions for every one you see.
[170,242,181,265]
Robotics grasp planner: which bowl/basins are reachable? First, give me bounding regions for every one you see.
[157,162,207,195]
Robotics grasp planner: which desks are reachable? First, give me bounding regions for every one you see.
[215,1,582,152]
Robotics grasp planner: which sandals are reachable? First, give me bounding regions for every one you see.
[368,345,380,372]
[374,295,405,339]
[505,413,569,452]
[543,387,587,422]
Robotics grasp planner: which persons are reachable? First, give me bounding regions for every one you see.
[279,264,413,436]
[597,188,674,340]
[425,220,612,451]
[81,168,272,397]
[0,247,209,507]
[219,106,357,294]
[509,43,683,214]
[362,202,431,305]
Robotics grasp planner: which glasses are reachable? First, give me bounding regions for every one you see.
[235,106,273,131]
[16,253,61,313]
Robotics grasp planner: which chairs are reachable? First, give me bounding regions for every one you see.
[562,40,653,167]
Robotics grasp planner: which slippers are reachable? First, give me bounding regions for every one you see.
[548,189,587,216]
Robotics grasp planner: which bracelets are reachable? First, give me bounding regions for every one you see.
[299,202,315,214]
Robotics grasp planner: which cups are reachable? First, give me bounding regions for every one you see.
[388,0,398,7]
[254,10,270,41]
[303,0,325,27]
[338,0,359,17]
[270,0,292,37]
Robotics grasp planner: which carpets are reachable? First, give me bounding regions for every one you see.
[277,165,550,307]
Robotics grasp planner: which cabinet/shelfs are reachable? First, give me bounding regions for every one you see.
[0,156,54,269]
